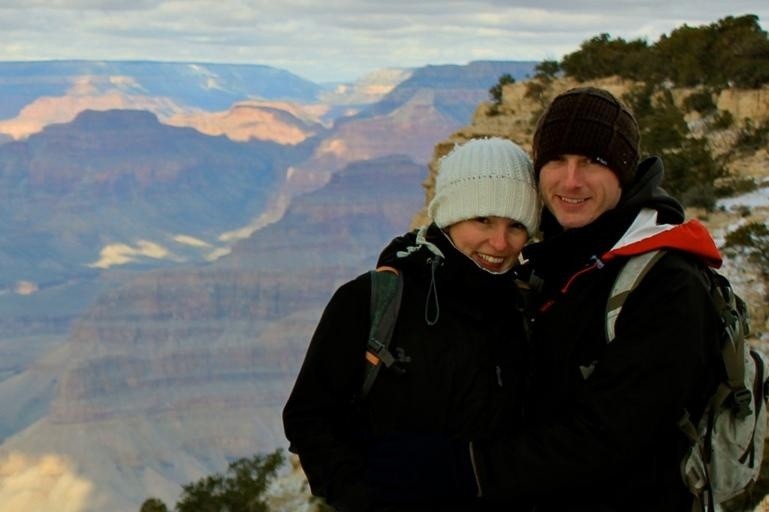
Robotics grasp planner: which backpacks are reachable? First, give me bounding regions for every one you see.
[606,248,769,511]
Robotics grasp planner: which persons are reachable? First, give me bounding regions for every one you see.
[521,84,723,510]
[282,138,542,512]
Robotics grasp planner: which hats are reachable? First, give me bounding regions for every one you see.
[428,136,544,238]
[534,86,641,183]
